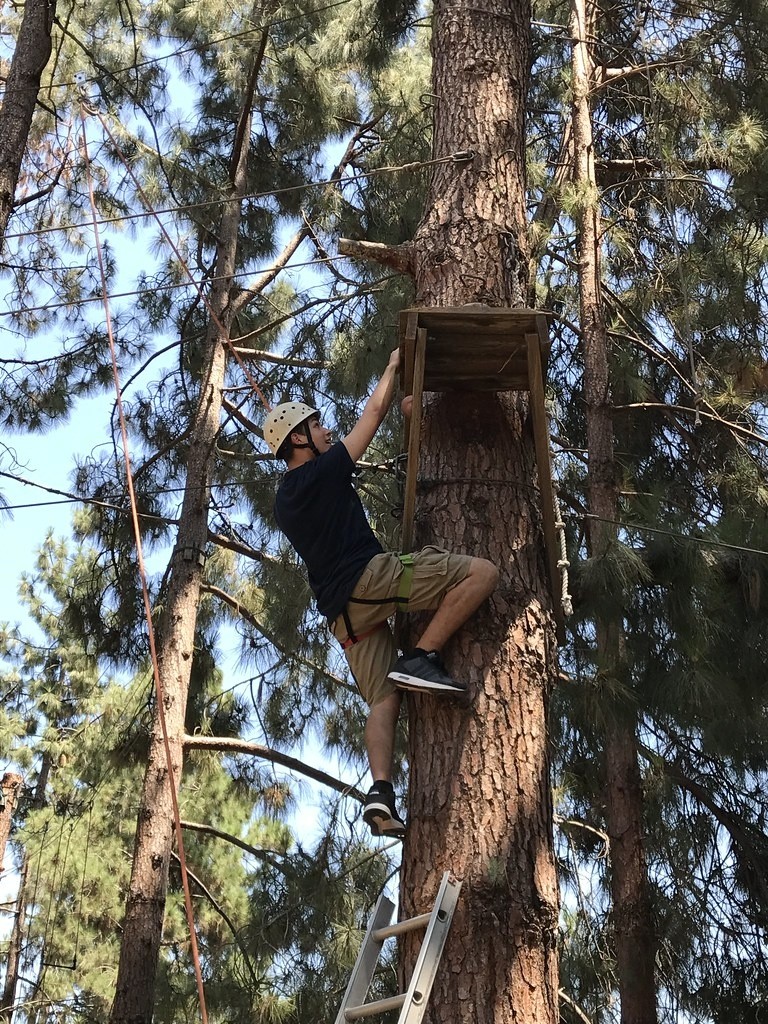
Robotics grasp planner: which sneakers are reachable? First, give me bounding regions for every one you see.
[387,647,467,693]
[363,788,406,836]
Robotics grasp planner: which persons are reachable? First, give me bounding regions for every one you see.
[263,349,501,834]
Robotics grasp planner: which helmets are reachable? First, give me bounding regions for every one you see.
[264,402,320,458]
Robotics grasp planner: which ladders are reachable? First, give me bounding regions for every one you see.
[331,869,464,1024]
